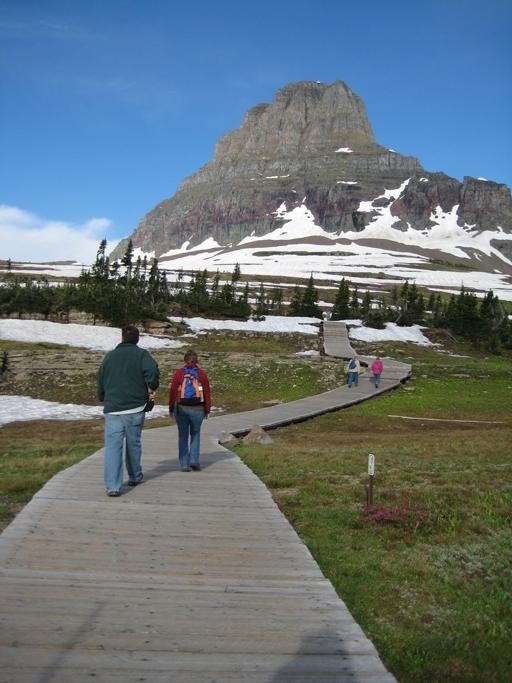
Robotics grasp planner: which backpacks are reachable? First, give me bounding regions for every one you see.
[349,359,356,369]
[177,366,205,404]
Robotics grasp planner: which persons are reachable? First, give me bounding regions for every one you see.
[371,355,383,388]
[167,348,212,472]
[96,324,160,497]
[346,354,361,389]
